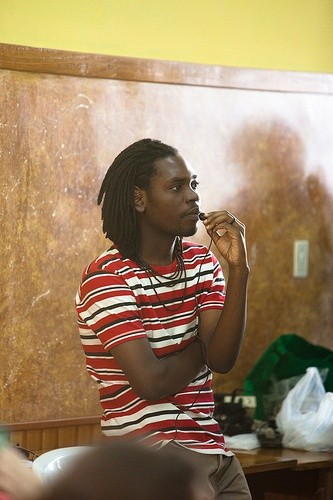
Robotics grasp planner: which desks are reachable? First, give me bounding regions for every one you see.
[227,446,333,500]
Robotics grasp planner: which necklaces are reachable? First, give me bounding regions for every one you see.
[131,247,186,312]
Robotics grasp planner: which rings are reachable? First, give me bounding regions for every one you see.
[230,217,235,224]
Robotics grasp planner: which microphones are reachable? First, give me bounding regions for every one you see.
[199,212,207,221]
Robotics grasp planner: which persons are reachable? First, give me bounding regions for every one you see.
[74,138,252,500]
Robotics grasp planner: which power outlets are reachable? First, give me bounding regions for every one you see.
[293,239,309,279]
